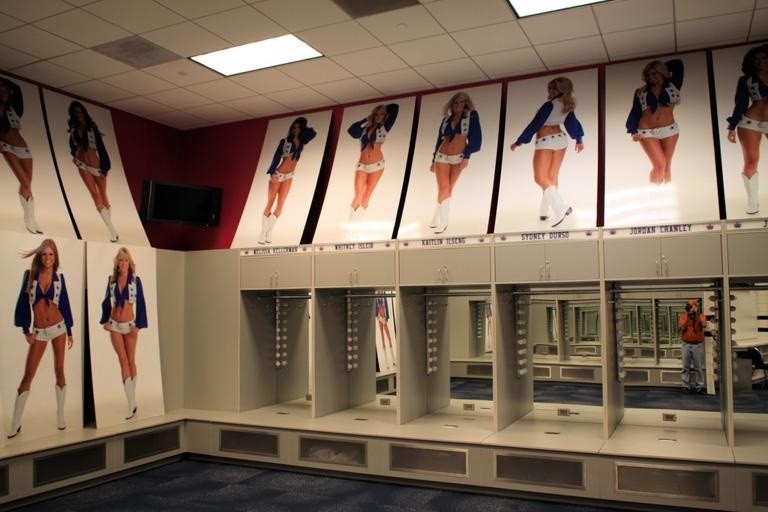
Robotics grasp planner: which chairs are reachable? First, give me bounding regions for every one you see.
[747,348,768,392]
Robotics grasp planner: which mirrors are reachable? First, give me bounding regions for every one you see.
[423,287,493,417]
[615,281,723,431]
[730,280,768,433]
[275,291,312,406]
[345,288,397,413]
[511,284,602,425]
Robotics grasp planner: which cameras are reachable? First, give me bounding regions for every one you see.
[685,303,696,319]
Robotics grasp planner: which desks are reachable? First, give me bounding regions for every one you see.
[732,338,768,392]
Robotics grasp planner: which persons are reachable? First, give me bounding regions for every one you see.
[511,77,584,228]
[678,298,707,394]
[347,103,400,212]
[9,238,74,438]
[428,92,482,234]
[100,247,148,419]
[627,59,685,184]
[67,101,121,243]
[728,46,768,215]
[375,291,397,368]
[1,77,43,233]
[258,117,317,244]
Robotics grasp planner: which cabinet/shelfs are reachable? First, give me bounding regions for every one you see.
[448,289,492,380]
[184,228,768,512]
[376,372,397,395]
[0,417,187,512]
[529,288,602,385]
[620,284,706,388]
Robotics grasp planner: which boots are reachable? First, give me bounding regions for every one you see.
[17,191,37,234]
[104,204,113,238]
[99,207,119,242]
[383,347,390,369]
[266,213,279,244]
[743,170,762,215]
[434,199,452,234]
[54,384,69,431]
[389,346,396,366]
[346,203,370,240]
[646,182,677,221]
[539,193,552,221]
[429,199,443,229]
[543,186,574,230]
[31,197,44,234]
[257,211,269,245]
[131,375,138,408]
[7,390,31,439]
[122,376,138,420]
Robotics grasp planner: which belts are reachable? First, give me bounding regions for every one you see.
[684,341,703,345]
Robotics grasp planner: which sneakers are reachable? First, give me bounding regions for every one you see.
[683,387,690,393]
[696,388,705,394]
